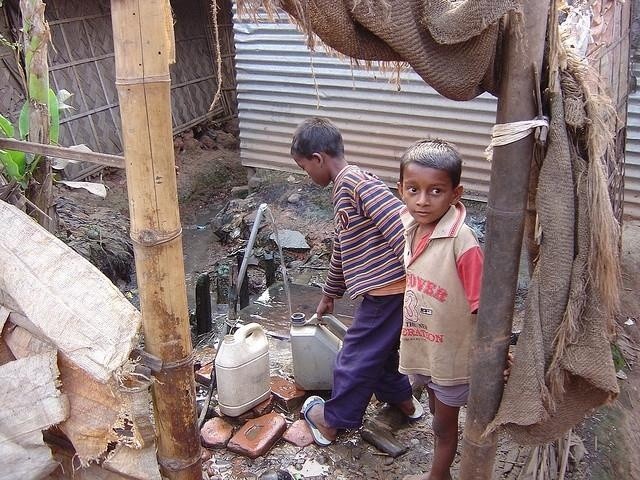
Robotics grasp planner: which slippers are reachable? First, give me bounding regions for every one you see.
[408,393,424,420]
[303,394,334,447]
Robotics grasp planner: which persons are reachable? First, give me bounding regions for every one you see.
[398,138,513,480]
[290,118,426,446]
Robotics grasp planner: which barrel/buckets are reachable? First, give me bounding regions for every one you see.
[289,312,348,391]
[214,322,272,417]
